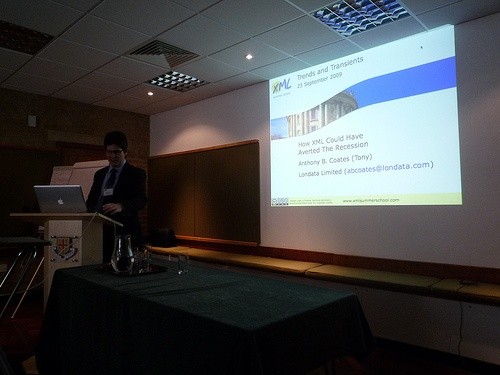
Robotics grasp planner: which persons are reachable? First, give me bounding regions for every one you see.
[85,131,146,264]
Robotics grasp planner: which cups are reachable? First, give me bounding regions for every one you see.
[177,252,189,275]
[136,247,150,274]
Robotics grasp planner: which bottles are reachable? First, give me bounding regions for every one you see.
[111,235,135,273]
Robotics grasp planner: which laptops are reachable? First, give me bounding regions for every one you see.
[32,184,89,213]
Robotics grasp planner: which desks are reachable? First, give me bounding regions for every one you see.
[34,257,376,375]
[0,237,52,319]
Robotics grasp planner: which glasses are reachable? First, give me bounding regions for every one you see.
[104,149,124,156]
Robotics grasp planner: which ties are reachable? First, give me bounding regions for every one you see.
[94,169,118,212]
